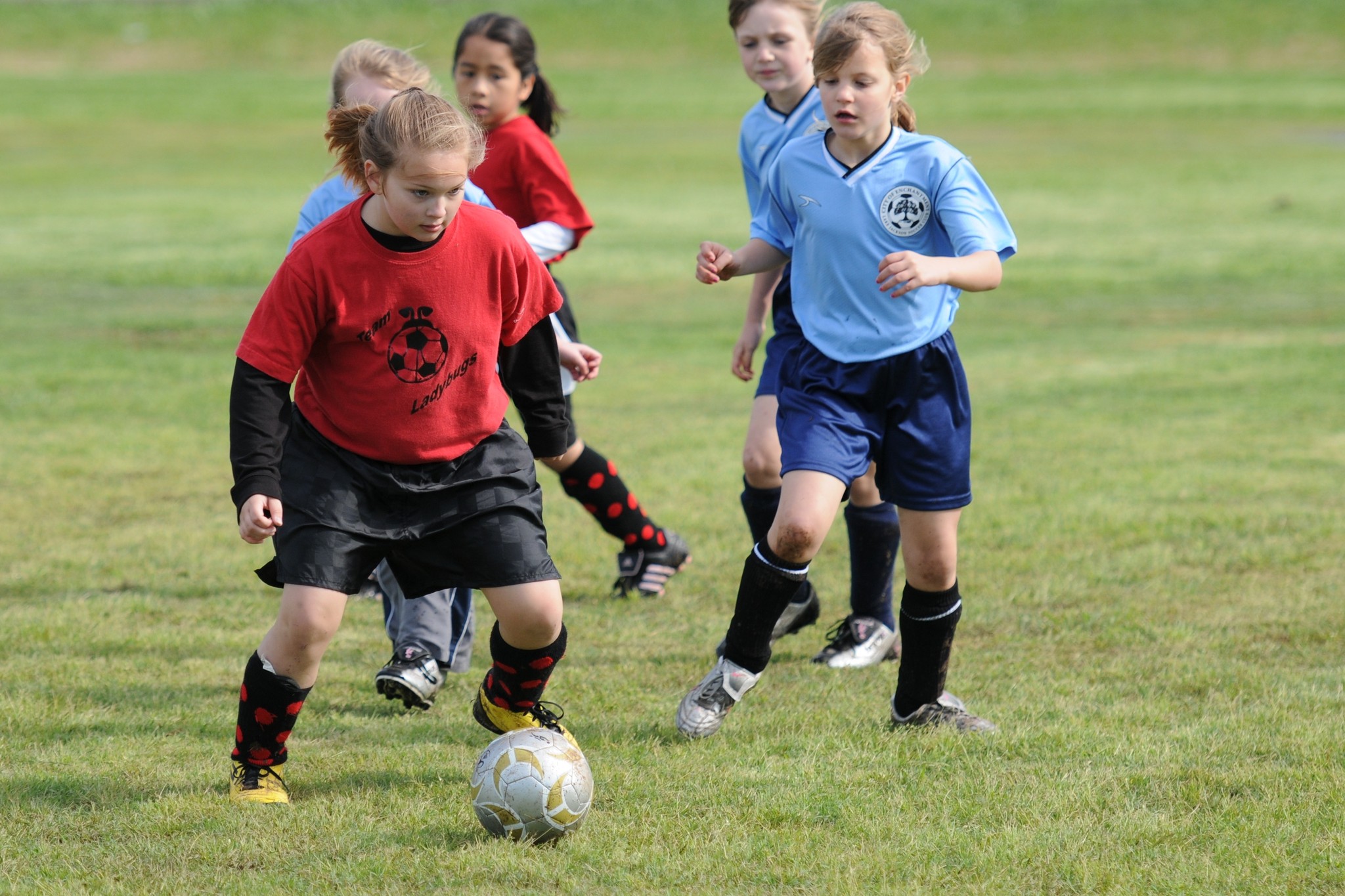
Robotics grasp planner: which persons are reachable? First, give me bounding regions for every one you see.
[729,0,898,669]
[229,87,570,804]
[677,0,1016,738]
[453,14,694,599]
[287,39,601,710]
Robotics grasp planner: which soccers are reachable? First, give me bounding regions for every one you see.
[469,727,594,846]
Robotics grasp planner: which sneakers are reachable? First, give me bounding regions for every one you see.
[814,617,899,667]
[374,647,442,709]
[611,528,692,603]
[674,659,765,739]
[472,683,576,746]
[229,752,289,804]
[888,691,995,734]
[716,581,818,656]
